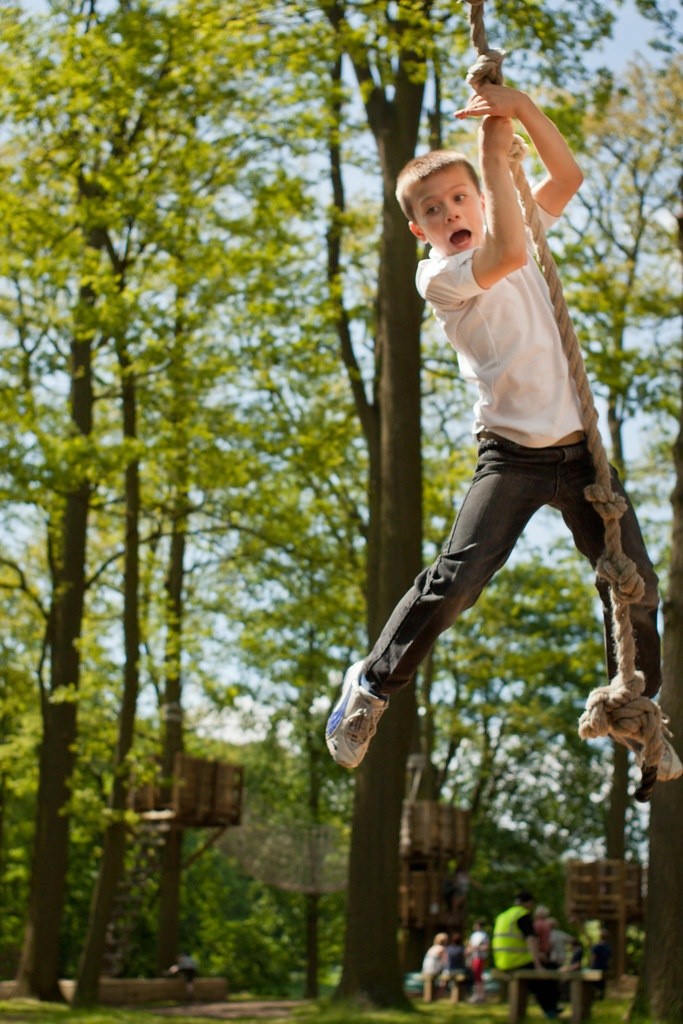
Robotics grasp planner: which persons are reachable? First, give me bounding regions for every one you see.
[178,948,199,983]
[323,79,682,783]
[421,903,619,1005]
[492,891,566,1024]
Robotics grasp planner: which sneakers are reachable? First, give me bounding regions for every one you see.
[325,659,391,769]
[612,726,683,782]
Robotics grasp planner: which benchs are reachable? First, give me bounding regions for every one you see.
[402,972,498,1001]
[495,968,603,1021]
